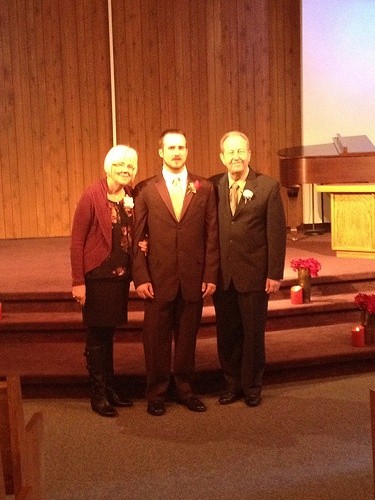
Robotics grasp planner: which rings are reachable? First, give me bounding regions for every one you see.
[73,296,75,298]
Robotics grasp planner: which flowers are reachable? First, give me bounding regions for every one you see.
[289,257,322,278]
[123,194,135,211]
[354,292,375,317]
[187,180,201,195]
[242,189,254,204]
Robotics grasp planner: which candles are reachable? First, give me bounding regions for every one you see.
[352,325,364,348]
[291,286,303,305]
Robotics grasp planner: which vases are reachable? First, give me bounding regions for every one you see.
[297,267,311,301]
[362,309,375,346]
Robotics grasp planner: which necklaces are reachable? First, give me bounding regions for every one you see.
[109,195,119,205]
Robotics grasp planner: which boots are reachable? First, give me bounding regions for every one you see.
[84,344,117,415]
[105,345,132,406]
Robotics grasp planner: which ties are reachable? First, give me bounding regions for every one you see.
[173,177,180,192]
[231,184,239,216]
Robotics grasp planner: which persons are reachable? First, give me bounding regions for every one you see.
[69,144,149,417]
[207,130,287,407]
[130,128,220,417]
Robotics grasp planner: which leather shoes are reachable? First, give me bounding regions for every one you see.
[147,402,165,415]
[245,393,261,406]
[220,391,238,404]
[176,397,207,412]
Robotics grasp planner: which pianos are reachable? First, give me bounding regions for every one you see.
[277,133,375,232]
